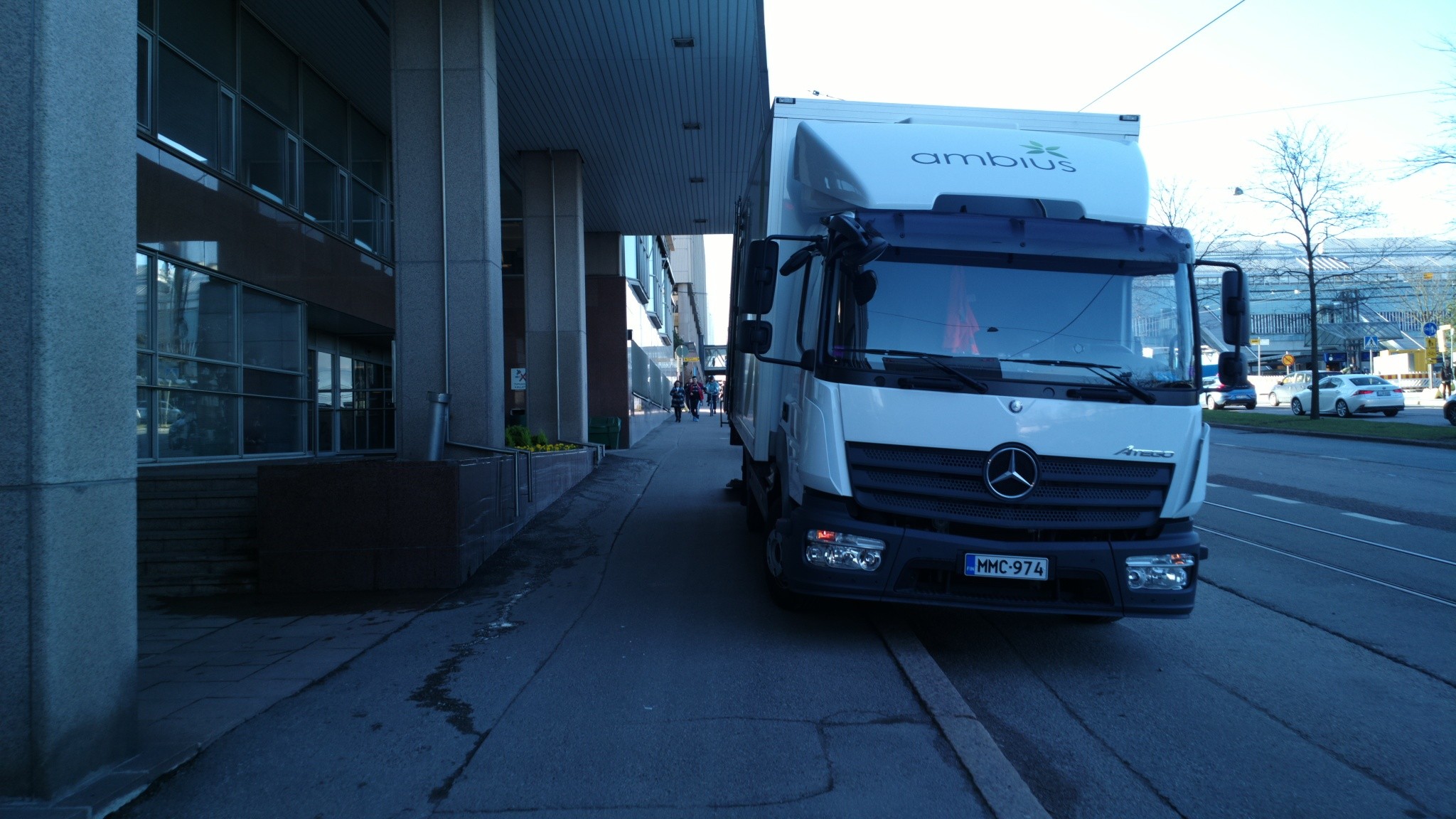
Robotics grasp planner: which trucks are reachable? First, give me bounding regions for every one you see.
[721,96,1252,621]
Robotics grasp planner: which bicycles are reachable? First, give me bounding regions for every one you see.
[703,390,720,417]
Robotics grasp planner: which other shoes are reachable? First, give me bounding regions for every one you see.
[694,416,699,422]
[676,418,678,422]
[679,418,680,422]
[708,402,709,406]
[714,410,716,414]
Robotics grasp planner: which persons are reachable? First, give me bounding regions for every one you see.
[169,395,196,449]
[704,375,719,414]
[687,376,704,421]
[669,380,685,423]
[683,377,692,413]
[1440,360,1454,400]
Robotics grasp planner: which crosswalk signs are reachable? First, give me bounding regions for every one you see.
[1363,336,1379,350]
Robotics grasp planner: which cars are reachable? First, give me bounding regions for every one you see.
[1199,376,1258,410]
[1289,372,1405,418]
[1267,369,1343,406]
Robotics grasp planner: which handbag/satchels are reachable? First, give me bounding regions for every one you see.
[1450,381,1455,391]
